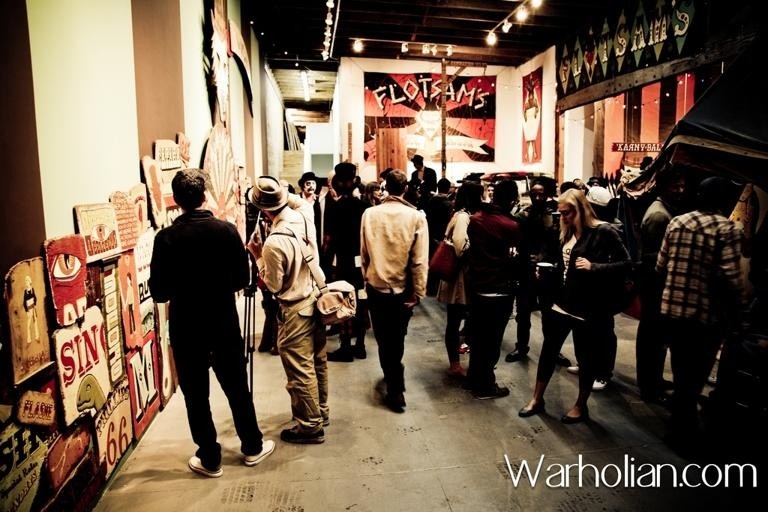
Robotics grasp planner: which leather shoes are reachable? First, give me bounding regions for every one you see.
[518,399,546,418]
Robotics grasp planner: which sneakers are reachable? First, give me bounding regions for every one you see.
[280,408,331,445]
[381,393,406,408]
[244,440,276,467]
[446,363,510,400]
[188,455,224,479]
[505,346,529,363]
[327,344,368,362]
[542,347,613,390]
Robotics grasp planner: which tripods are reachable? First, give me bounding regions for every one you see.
[243,217,278,405]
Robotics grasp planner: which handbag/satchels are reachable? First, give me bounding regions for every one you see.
[430,234,463,282]
[576,268,638,320]
[317,280,359,324]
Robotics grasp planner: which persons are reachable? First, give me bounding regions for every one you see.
[149,170,276,477]
[245,154,754,459]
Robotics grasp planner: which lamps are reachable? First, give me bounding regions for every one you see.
[291,1,544,105]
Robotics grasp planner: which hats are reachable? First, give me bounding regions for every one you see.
[332,163,361,191]
[411,154,424,165]
[587,176,599,185]
[247,176,288,213]
[298,172,319,184]
